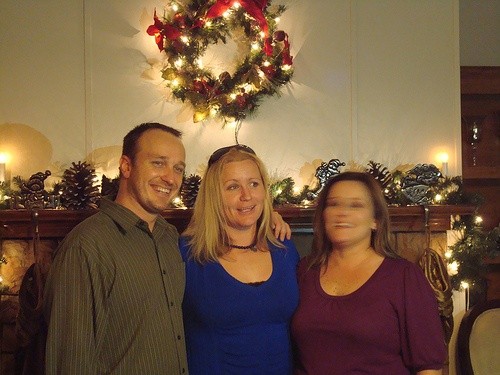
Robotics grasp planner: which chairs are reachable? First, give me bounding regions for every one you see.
[458,299,500,375]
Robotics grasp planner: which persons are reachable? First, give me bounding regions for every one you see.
[41,123,291,375]
[286,171,447,375]
[177,144,300,375]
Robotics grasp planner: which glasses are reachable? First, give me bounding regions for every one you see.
[208,145,255,169]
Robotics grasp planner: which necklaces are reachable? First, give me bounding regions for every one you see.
[225,234,257,253]
